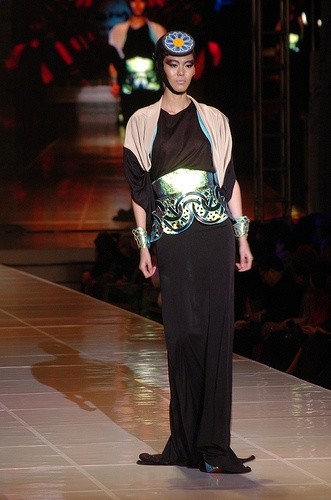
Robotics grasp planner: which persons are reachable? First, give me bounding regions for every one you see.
[121,29,257,475]
[107,0,167,224]
[79,207,330,389]
